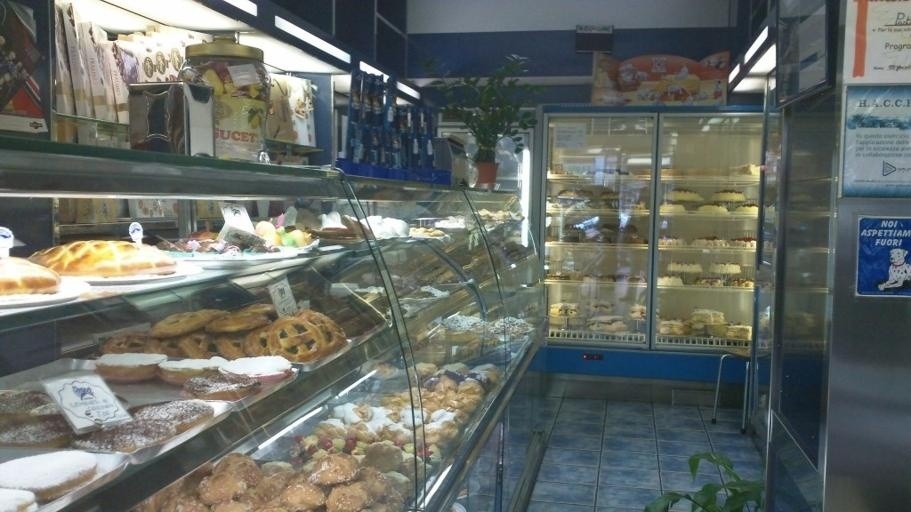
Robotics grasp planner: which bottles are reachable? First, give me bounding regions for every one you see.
[351,71,402,171]
[404,105,436,168]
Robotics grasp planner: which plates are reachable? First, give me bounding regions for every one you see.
[0,249,297,309]
[318,235,368,246]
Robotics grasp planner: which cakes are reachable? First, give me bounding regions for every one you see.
[549,163,759,341]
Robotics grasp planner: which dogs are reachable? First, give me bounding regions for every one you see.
[875,247,911,291]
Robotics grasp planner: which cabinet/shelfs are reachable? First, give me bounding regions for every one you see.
[516,85,784,396]
[3,1,553,510]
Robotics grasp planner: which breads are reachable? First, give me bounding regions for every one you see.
[0,209,538,512]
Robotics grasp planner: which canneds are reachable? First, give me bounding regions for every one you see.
[177,39,272,163]
